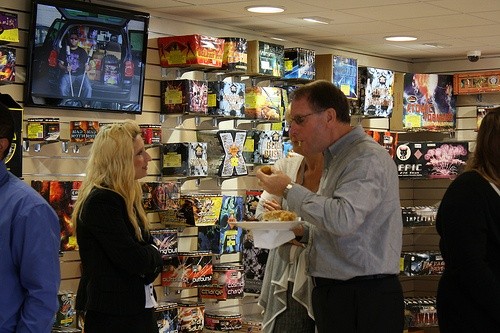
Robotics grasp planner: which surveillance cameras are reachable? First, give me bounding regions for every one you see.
[467,51,481,62]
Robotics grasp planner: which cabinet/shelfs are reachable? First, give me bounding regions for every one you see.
[139,63,500,333]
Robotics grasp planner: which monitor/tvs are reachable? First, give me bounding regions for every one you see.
[23,0,150,115]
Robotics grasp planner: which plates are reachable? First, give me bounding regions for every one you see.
[228,221,300,230]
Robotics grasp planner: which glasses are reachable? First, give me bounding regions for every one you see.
[290,109,328,125]
[70,37,78,41]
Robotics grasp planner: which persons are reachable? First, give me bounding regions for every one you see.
[255,102,320,333]
[436,107,500,333]
[57,34,94,99]
[0,92,60,333]
[257,80,406,333]
[70,121,167,333]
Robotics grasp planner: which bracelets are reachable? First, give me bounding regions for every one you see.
[283,181,297,200]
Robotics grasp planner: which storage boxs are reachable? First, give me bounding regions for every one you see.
[159,140,208,177]
[402,251,446,277]
[156,33,359,118]
[395,141,469,179]
[402,206,437,227]
[405,295,439,326]
